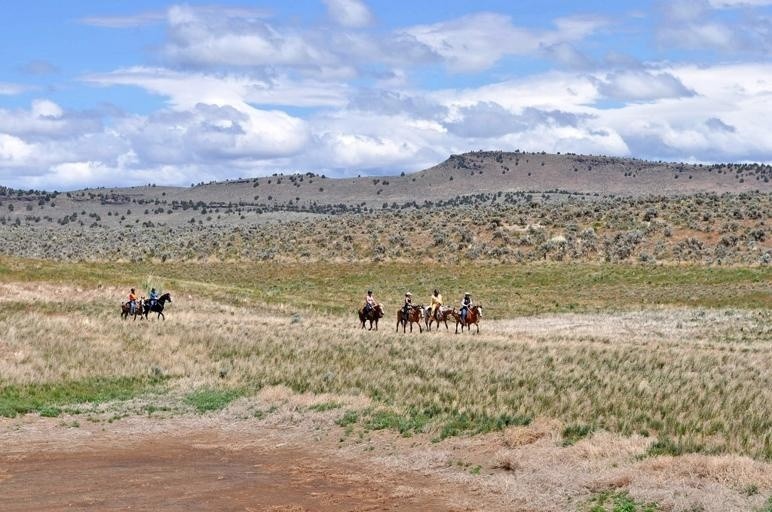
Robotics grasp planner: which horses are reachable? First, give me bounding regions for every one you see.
[120,295,145,321]
[396,304,425,333]
[358,302,384,331]
[424,305,459,333]
[455,305,482,334]
[143,293,172,321]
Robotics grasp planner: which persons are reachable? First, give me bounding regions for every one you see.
[428,288,444,321]
[140,295,147,313]
[460,291,473,325]
[364,289,377,319]
[130,287,139,315]
[148,287,158,313]
[403,290,414,319]
